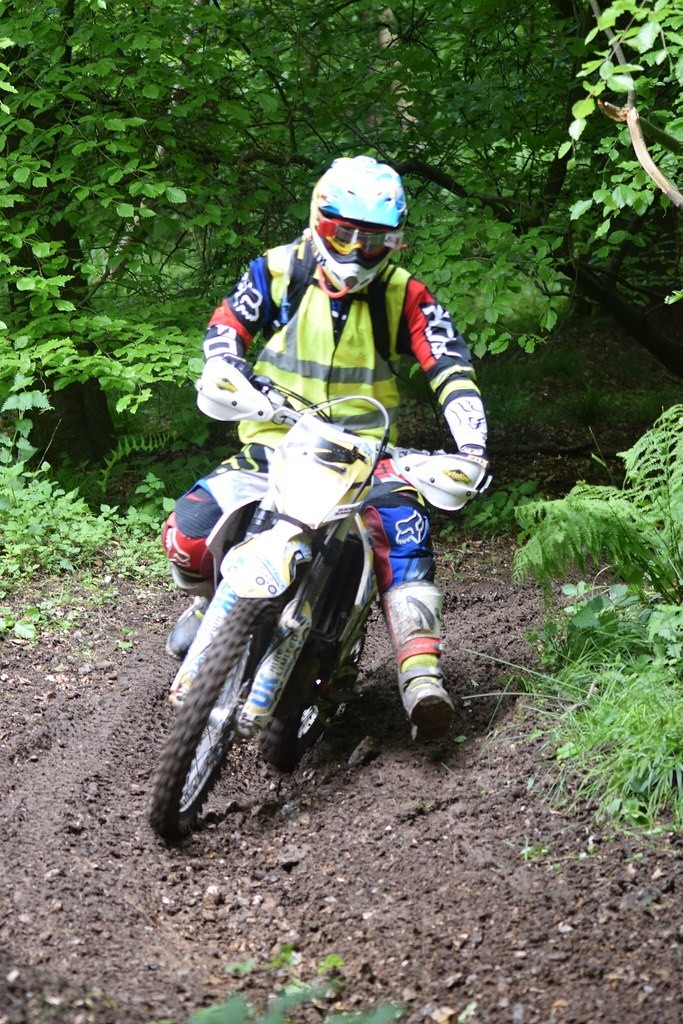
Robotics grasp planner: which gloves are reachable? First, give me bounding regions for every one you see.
[457,444,490,489]
[213,353,253,378]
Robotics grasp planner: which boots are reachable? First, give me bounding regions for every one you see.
[380,583,455,741]
[165,568,217,660]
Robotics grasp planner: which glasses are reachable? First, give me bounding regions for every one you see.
[317,212,398,256]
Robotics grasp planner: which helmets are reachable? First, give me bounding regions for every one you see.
[309,155,407,293]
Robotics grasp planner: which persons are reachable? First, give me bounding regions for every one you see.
[161,156,492,743]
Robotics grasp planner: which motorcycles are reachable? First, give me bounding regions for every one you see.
[148,353,493,847]
